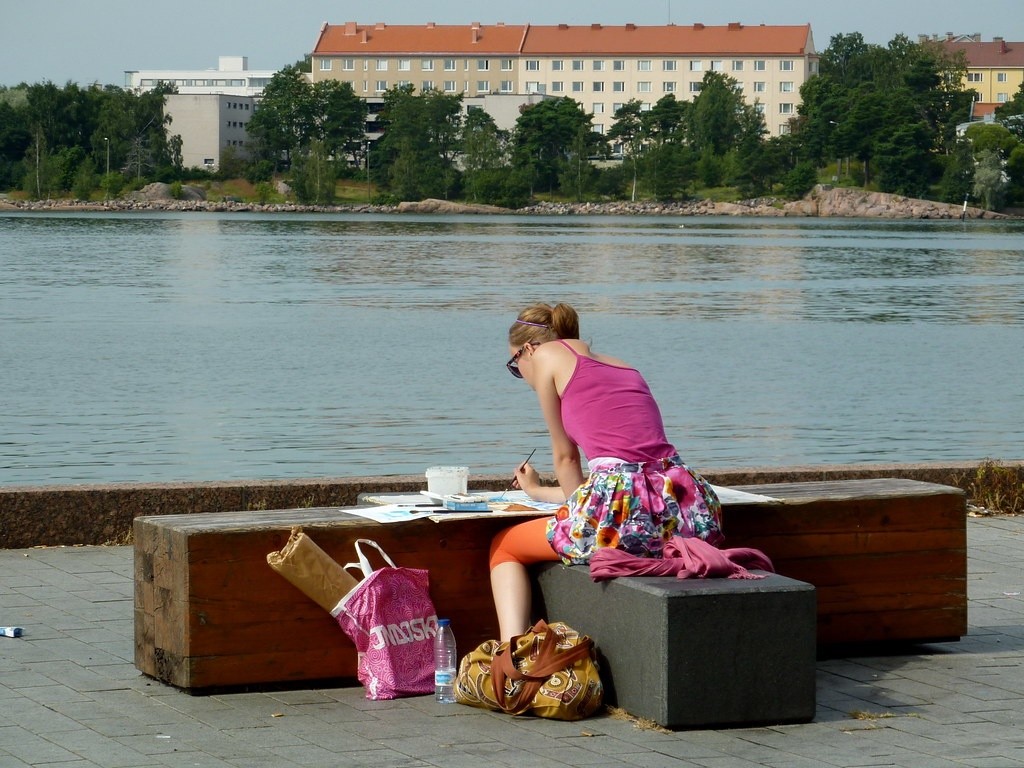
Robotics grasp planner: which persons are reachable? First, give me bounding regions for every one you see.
[488,306,722,645]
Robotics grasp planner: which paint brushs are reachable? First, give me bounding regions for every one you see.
[397,504,444,507]
[409,509,494,514]
[498,449,537,500]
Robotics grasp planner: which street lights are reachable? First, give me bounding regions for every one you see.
[364,141,371,201]
[104,137,111,178]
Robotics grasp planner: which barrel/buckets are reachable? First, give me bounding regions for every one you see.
[426,466,468,497]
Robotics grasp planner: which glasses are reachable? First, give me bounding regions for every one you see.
[506,342,539,379]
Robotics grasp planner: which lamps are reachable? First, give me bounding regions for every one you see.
[104,138,108,140]
[367,141,371,145]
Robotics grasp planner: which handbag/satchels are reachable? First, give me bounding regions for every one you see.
[452,620,603,722]
[328,537,441,700]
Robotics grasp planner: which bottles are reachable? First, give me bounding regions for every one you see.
[434,620,456,704]
